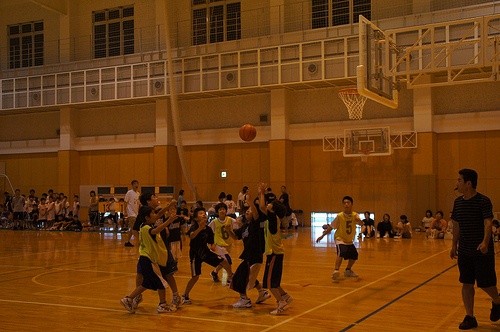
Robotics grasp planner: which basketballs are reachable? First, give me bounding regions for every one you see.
[239,124,256,141]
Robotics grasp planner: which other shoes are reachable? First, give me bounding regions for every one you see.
[357,233,362,237]
[331,270,340,280]
[377,233,380,238]
[344,270,358,277]
[383,234,389,238]
[125,242,134,247]
[367,234,370,238]
[459,315,478,330]
[490,293,500,322]
[394,233,402,239]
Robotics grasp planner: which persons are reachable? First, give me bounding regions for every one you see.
[449,168,500,329]
[422,208,456,241]
[490,220,500,241]
[0,179,300,315]
[316,195,365,280]
[395,215,413,239]
[376,214,394,239]
[357,210,375,239]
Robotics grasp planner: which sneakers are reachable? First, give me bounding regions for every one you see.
[210,270,219,282]
[256,289,271,304]
[233,297,252,308]
[181,295,192,304]
[132,293,143,309]
[172,296,181,306]
[120,296,135,314]
[269,300,289,315]
[226,279,232,286]
[281,293,295,306]
[157,303,173,312]
